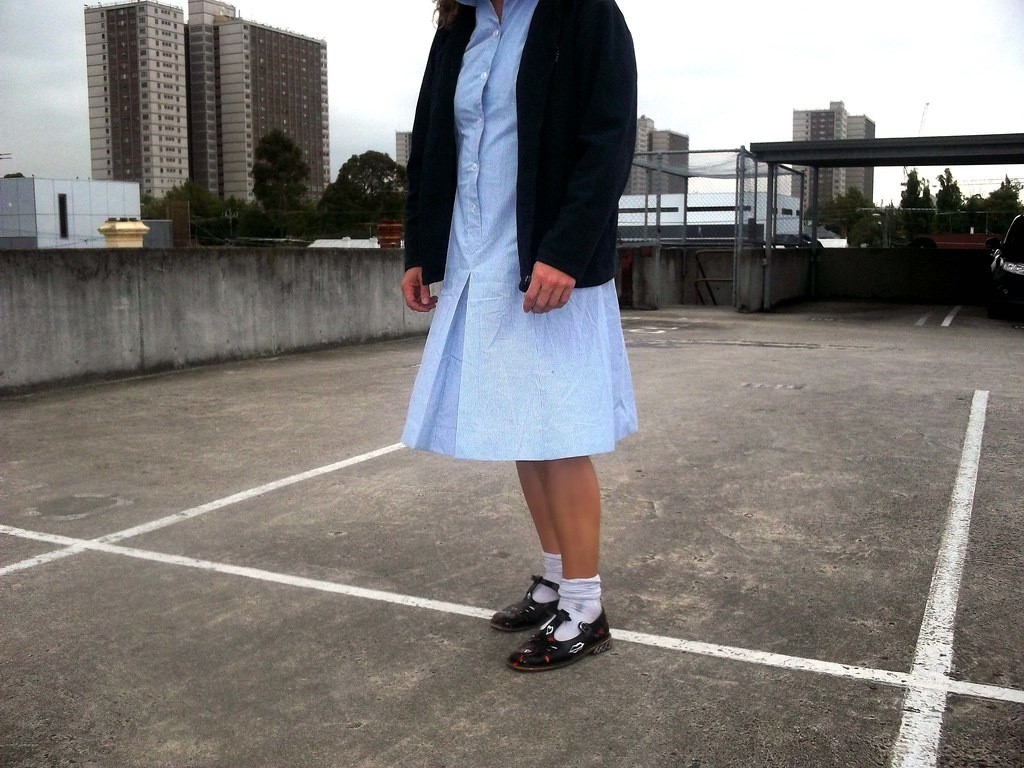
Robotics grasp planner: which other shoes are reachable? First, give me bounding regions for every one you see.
[489,575,560,632]
[506,605,612,673]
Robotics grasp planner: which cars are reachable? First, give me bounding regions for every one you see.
[983,213,1024,320]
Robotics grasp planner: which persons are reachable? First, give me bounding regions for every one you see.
[400,1,643,673]
